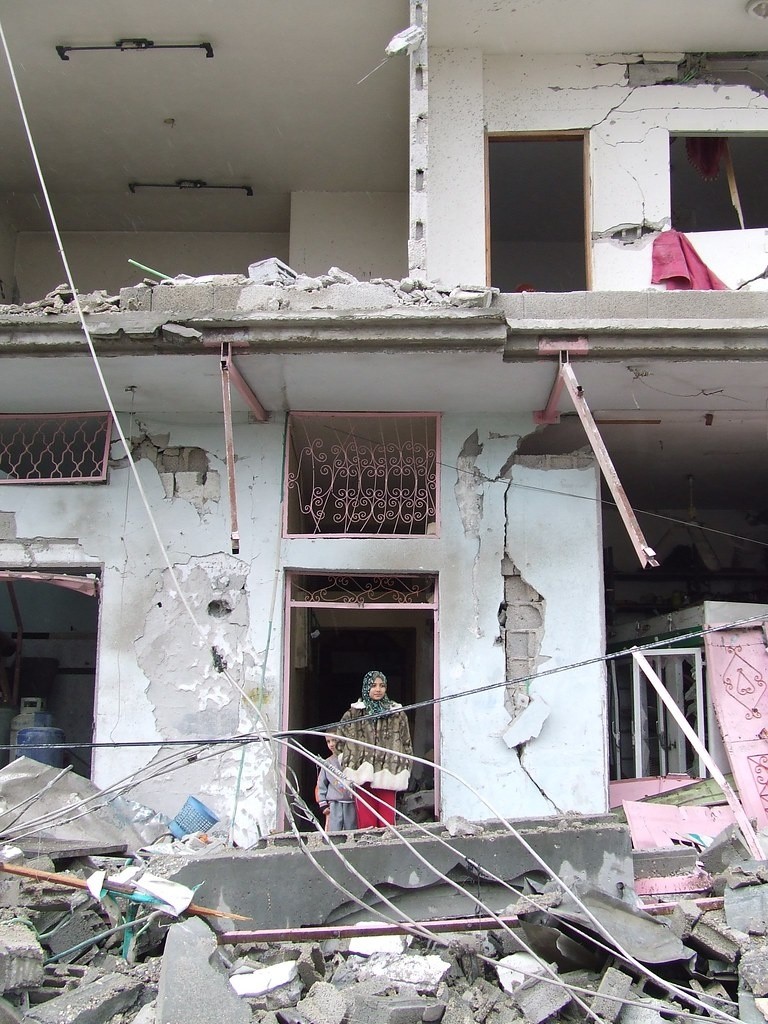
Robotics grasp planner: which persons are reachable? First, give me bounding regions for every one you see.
[314,726,356,832]
[336,671,414,829]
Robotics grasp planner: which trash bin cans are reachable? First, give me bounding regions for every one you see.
[168,795,220,840]
